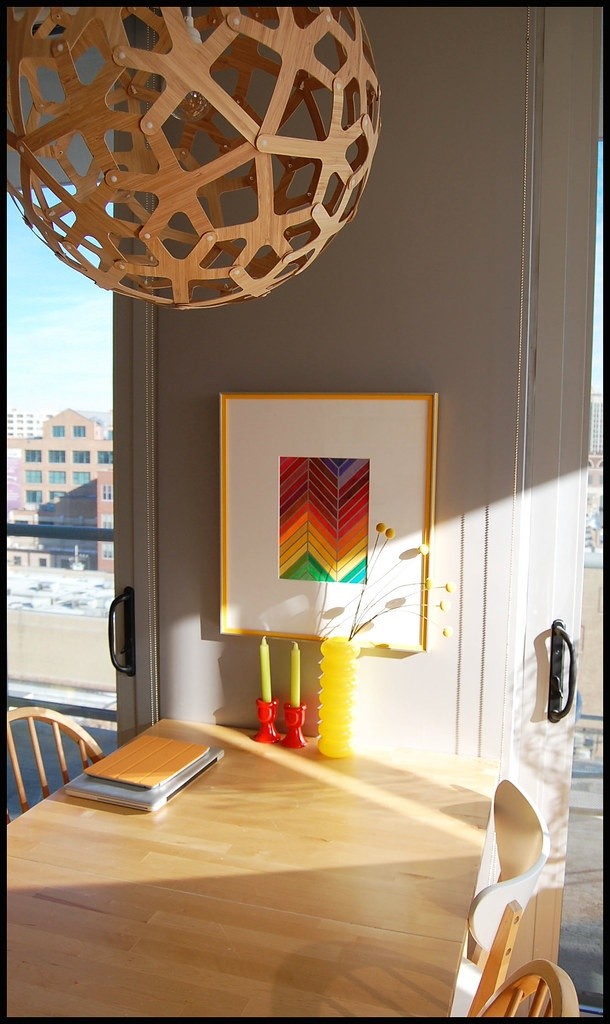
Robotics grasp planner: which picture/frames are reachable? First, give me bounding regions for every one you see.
[219,392,439,652]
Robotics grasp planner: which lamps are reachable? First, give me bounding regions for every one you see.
[5,3,384,308]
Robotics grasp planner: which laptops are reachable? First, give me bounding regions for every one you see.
[65,742,224,811]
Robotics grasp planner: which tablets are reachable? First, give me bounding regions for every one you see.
[84,736,211,790]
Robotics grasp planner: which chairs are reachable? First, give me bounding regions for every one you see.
[462,776,583,1018]
[7,708,103,823]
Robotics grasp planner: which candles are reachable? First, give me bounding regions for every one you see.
[260,636,272,703]
[289,642,301,706]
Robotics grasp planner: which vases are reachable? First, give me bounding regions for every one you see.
[317,638,358,756]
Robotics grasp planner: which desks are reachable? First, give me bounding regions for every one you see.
[7,719,491,1018]
